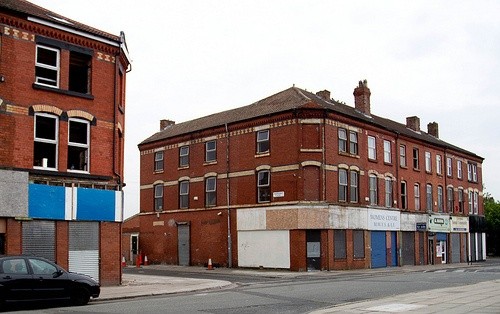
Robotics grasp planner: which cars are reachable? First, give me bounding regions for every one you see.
[0,254,100,309]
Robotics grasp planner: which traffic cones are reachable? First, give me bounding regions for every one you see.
[135,255,142,268]
[205,259,216,270]
[139,252,143,264]
[144,252,149,266]
[122,257,128,267]
